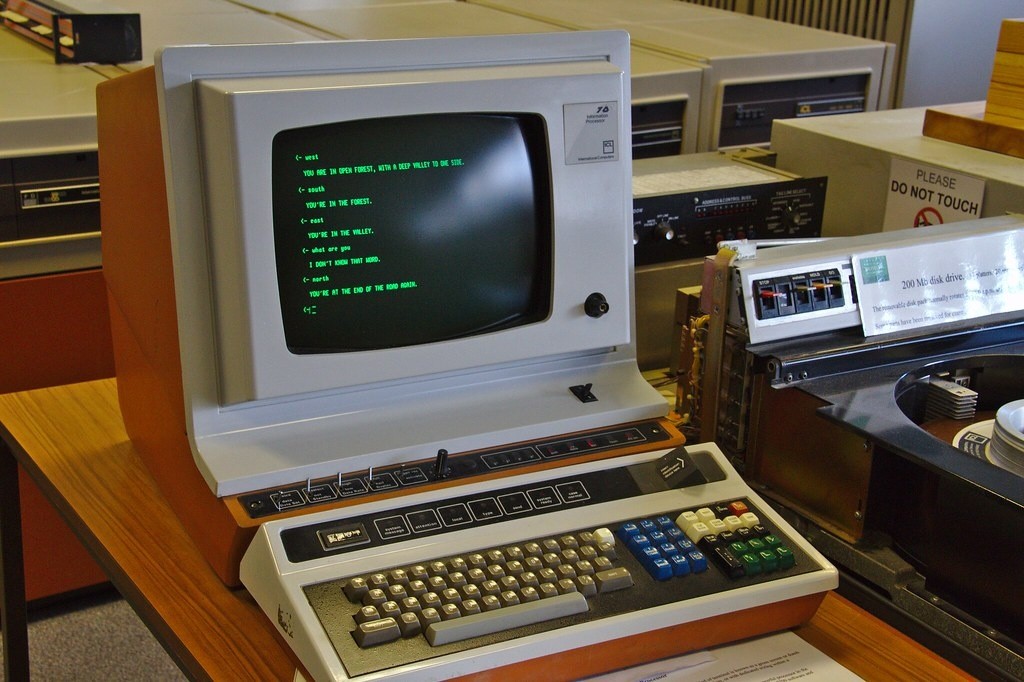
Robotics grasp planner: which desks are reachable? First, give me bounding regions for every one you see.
[0,377,978,682]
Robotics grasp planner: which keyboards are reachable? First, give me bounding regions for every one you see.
[240,443,838,682]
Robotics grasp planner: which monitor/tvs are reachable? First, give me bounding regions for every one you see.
[188,60,631,411]
